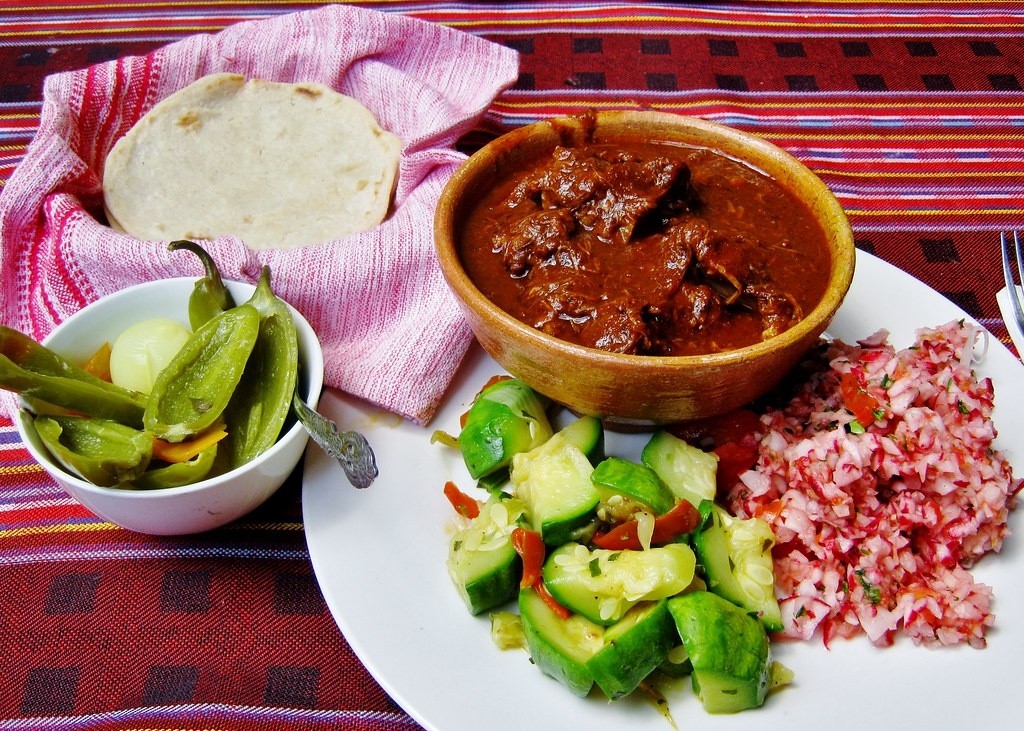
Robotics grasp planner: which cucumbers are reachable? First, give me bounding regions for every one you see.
[444,382,784,714]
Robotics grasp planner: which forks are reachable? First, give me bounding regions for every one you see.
[1000,230,1024,338]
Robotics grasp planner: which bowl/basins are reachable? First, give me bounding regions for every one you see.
[434,110,856,435]
[12,276,325,536]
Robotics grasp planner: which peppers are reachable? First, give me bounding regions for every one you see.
[0,240,298,488]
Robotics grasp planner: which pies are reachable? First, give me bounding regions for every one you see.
[100,69,400,250]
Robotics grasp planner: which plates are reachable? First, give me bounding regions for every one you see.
[302,248,1024,730]
